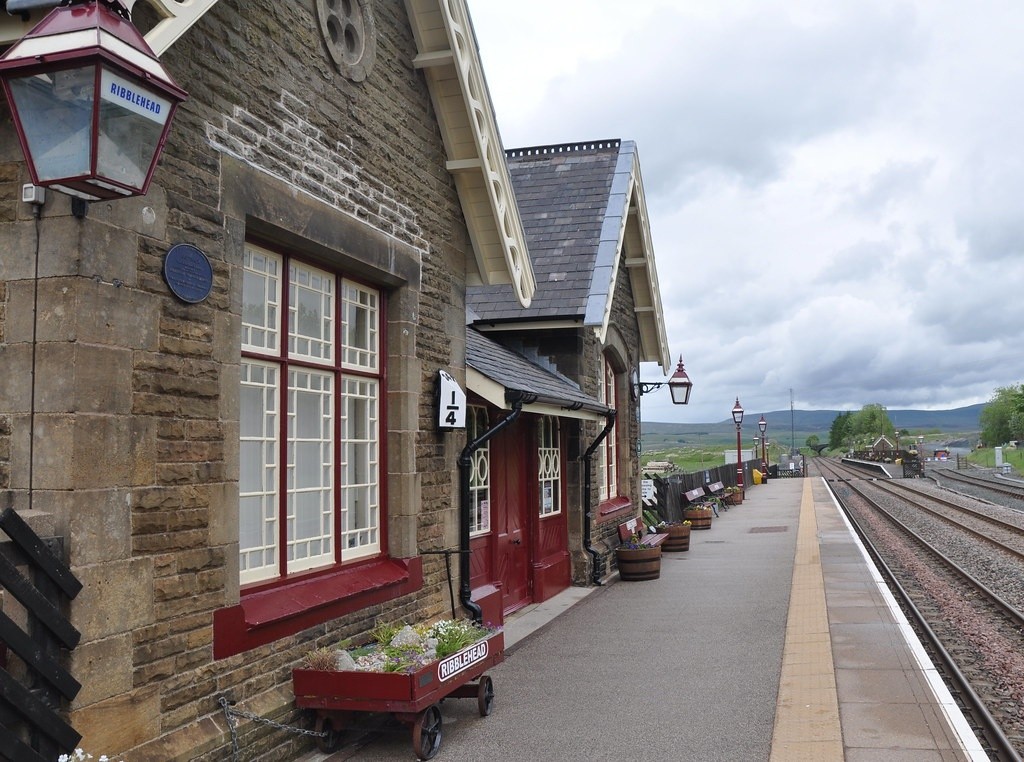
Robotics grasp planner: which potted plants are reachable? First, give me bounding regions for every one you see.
[706,496,720,514]
[724,486,743,505]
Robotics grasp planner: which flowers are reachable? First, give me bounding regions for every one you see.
[618,534,659,550]
[304,613,504,673]
[688,505,711,510]
[658,520,692,527]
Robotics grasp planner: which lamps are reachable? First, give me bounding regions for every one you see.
[639,354,694,404]
[0,0,189,220]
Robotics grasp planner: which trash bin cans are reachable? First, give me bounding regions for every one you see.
[752,470,763,486]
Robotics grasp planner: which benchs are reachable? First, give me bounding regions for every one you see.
[685,487,719,518]
[617,516,669,546]
[708,481,736,512]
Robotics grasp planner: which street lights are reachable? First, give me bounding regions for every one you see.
[894,431,900,460]
[731,395,747,501]
[862,438,867,455]
[757,413,767,484]
[765,441,770,466]
[870,437,876,452]
[881,434,886,457]
[852,439,856,454]
[753,433,759,459]
[856,440,861,455]
[918,434,924,458]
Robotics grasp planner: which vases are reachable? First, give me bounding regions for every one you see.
[615,547,661,581]
[656,526,690,552]
[292,630,503,714]
[683,510,712,530]
